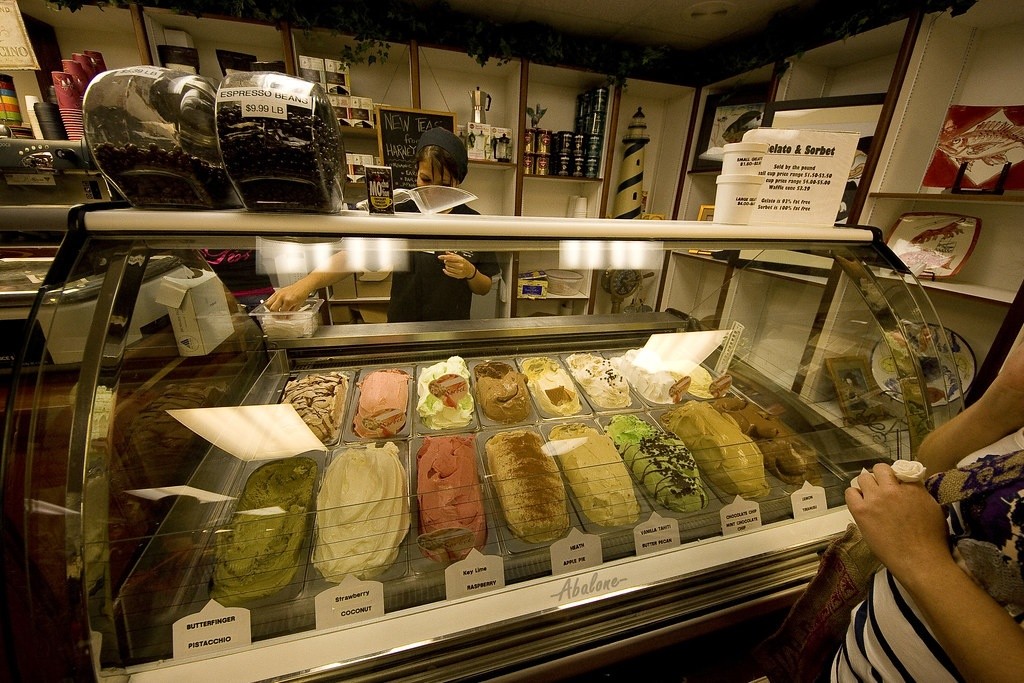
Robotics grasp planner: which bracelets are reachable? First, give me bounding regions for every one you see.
[466,267,477,280]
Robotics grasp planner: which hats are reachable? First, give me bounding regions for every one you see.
[416,127,468,184]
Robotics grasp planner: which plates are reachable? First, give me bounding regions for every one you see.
[872,322,976,407]
[881,210,983,281]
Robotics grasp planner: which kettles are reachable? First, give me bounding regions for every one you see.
[492,133,511,164]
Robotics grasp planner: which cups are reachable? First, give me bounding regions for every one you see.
[52,50,108,141]
[24,94,45,140]
[566,196,587,218]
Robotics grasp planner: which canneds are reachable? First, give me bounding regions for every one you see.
[524,86,610,178]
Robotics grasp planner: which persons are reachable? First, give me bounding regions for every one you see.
[262,127,503,322]
[824,321,1024,683]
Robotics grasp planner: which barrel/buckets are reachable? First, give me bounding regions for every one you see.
[713,173,765,224]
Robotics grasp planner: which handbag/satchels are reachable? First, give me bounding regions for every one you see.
[754,521,884,683]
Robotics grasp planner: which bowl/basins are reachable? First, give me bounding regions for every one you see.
[544,268,584,297]
[722,143,769,178]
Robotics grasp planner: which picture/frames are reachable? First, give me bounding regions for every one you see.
[823,355,889,427]
[692,88,768,171]
[688,204,723,255]
[728,92,888,277]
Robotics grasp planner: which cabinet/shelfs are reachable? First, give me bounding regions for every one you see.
[128,2,697,326]
[658,0,1024,461]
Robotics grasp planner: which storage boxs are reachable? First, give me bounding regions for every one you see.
[327,94,349,125]
[350,95,374,129]
[155,268,235,356]
[465,121,491,161]
[345,153,379,184]
[163,28,195,48]
[491,127,513,163]
[331,272,393,323]
[373,103,391,129]
[324,58,351,97]
[457,125,466,147]
[248,297,325,338]
[518,270,549,300]
[298,54,328,94]
